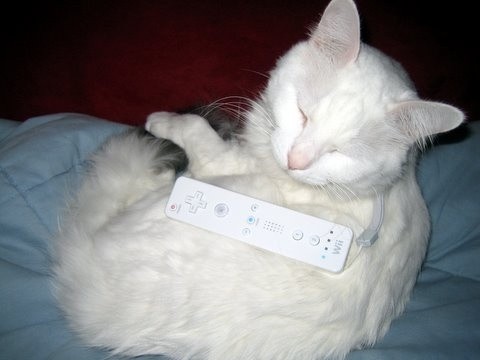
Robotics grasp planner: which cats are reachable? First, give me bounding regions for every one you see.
[45,0,465,360]
